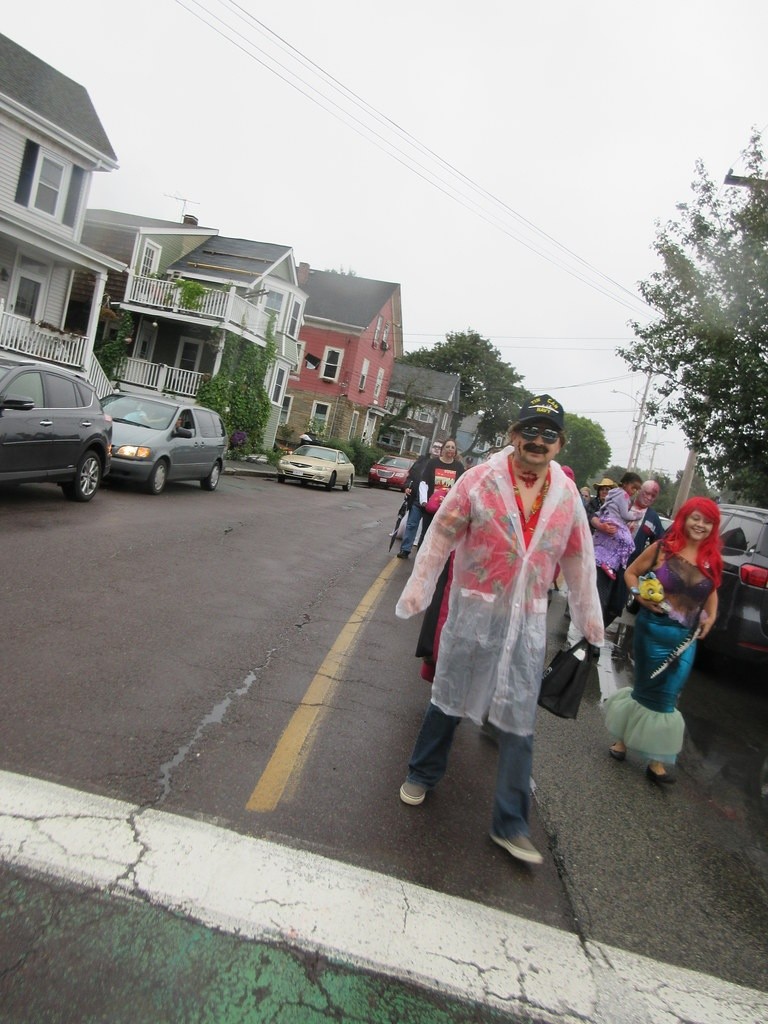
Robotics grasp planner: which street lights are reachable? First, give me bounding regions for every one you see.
[647,440,675,481]
[609,389,648,473]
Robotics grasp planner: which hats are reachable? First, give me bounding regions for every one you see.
[593,479,618,492]
[519,394,565,431]
[562,465,575,482]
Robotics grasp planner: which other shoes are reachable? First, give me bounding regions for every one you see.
[645,765,676,784]
[609,742,627,760]
[398,550,409,558]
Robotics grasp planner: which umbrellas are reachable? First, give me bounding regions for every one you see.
[389,481,413,551]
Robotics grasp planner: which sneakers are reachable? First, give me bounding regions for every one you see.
[399,779,426,805]
[490,827,544,864]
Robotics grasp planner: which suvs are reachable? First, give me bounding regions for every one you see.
[693,503,768,686]
[0,354,115,503]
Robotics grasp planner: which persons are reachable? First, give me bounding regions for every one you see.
[176,413,183,429]
[548,465,665,660]
[602,498,723,785]
[394,395,606,863]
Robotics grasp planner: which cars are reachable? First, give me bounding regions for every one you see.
[367,454,419,494]
[276,443,356,492]
[97,391,230,496]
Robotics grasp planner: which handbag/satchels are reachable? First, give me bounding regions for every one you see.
[538,638,596,720]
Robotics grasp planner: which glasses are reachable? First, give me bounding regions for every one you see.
[515,425,562,445]
[432,445,443,451]
